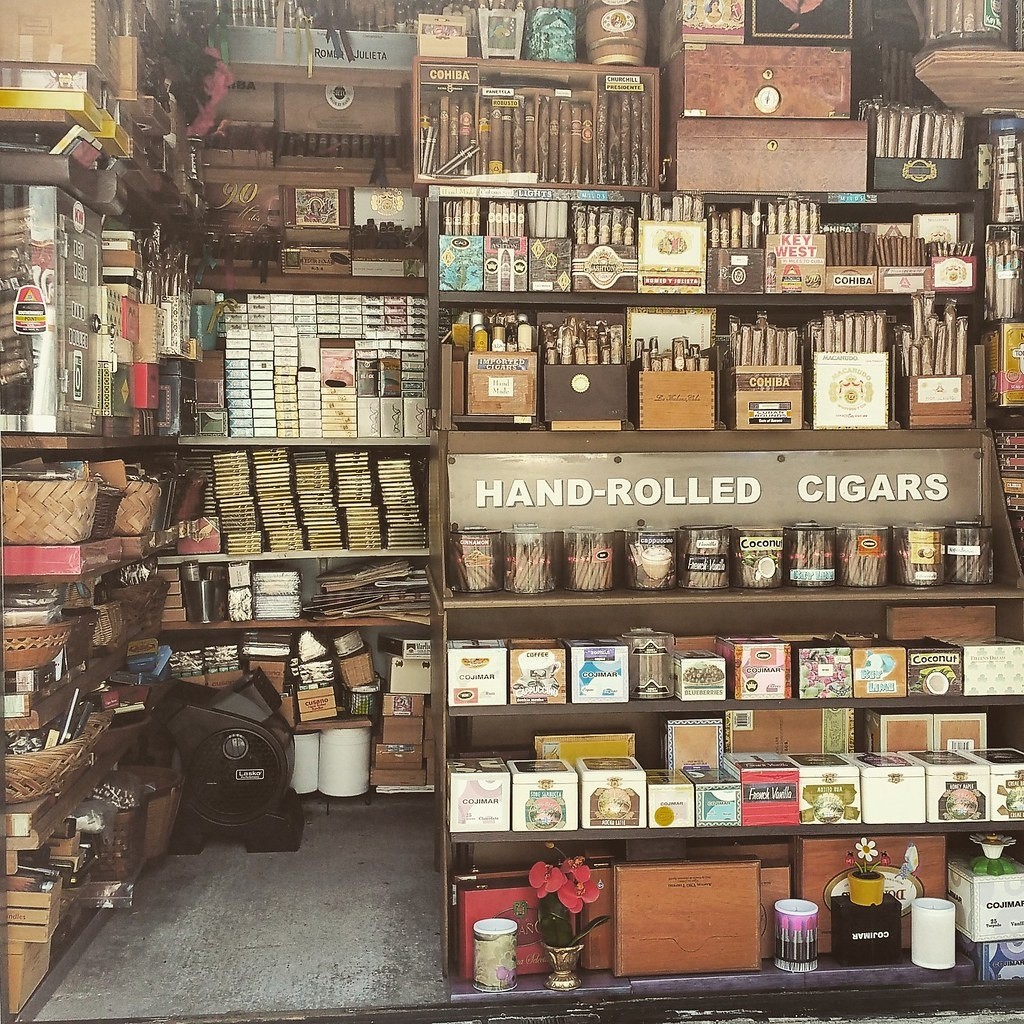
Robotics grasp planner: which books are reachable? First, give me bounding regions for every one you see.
[57,687,95,745]
[920,0,1002,42]
[142,446,428,556]
[248,557,432,627]
[912,210,960,250]
[241,628,294,662]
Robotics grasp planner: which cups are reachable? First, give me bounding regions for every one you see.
[911,898,955,967]
[774,899,820,971]
[474,918,521,992]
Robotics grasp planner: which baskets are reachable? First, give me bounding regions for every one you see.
[3,575,171,672]
[4,709,154,803]
[4,477,178,545]
[344,678,388,717]
[333,643,374,689]
[80,764,185,881]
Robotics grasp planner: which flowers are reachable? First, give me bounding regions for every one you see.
[845,838,890,872]
[529,842,611,947]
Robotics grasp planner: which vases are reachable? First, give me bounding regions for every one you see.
[540,941,585,992]
[848,871,884,906]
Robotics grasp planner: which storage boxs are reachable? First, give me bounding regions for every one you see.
[7,877,63,1014]
[0,0,172,135]
[447,606,1023,988]
[179,634,432,786]
[412,0,973,430]
[98,229,228,437]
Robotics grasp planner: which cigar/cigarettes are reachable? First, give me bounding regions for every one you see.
[0,205,34,386]
[880,33,934,103]
[444,520,998,593]
[419,87,653,188]
[440,192,975,267]
[983,228,1024,320]
[446,289,970,376]
[861,102,964,160]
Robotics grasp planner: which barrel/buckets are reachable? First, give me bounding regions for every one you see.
[585,0,647,66]
[319,730,370,797]
[184,579,227,623]
[290,733,319,793]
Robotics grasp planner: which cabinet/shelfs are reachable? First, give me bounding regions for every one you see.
[409,0,1024,1001]
[0,0,432,1023]
